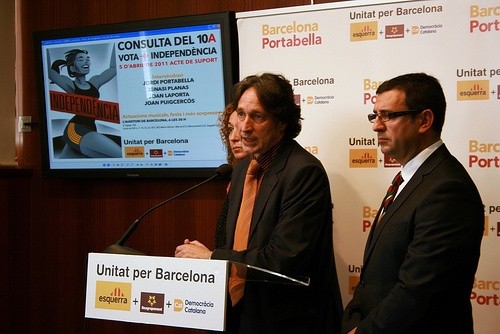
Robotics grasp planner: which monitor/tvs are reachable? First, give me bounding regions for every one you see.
[32,11,240,179]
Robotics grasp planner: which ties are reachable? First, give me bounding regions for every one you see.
[228,160,261,306]
[375,171,404,223]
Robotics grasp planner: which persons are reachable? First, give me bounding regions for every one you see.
[174,73,344,334]
[211,99,252,249]
[341,73,485,334]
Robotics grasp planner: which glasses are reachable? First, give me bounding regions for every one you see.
[368,110,411,123]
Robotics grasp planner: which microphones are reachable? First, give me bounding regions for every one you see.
[117,164,232,246]
